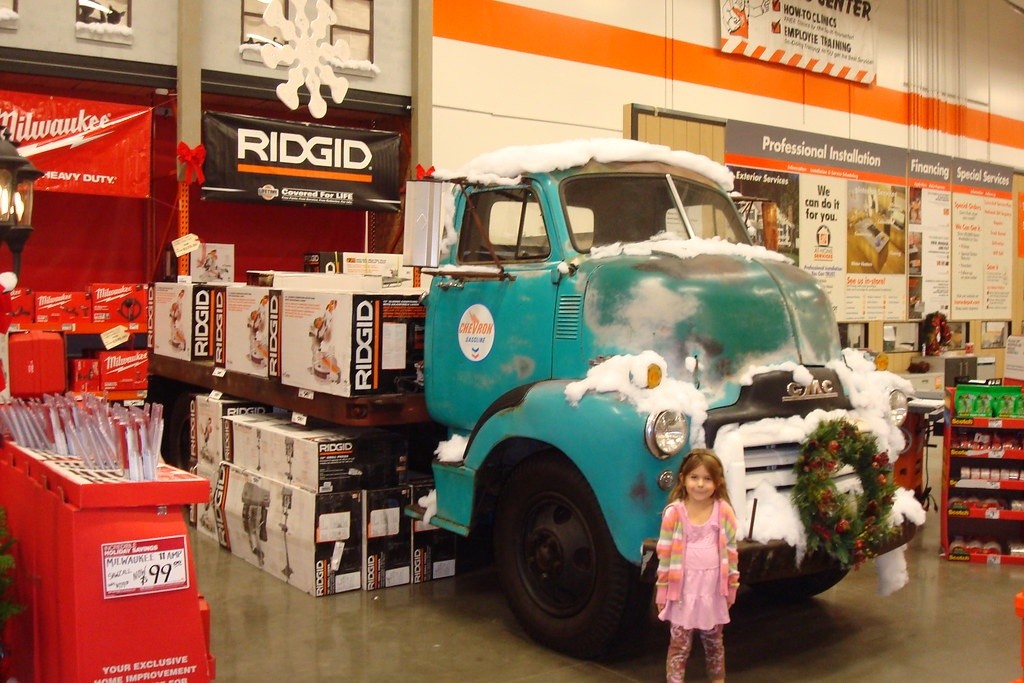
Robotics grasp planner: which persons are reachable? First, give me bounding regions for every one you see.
[205,250,228,278]
[311,301,341,385]
[248,295,269,363]
[655,450,741,683]
[169,290,186,351]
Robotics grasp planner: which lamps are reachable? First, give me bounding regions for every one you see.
[0,123,44,278]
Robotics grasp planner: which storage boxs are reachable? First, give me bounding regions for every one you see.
[0,243,460,600]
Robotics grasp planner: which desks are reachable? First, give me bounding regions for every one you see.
[149,353,431,469]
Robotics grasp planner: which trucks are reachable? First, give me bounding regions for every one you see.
[144,142,925,663]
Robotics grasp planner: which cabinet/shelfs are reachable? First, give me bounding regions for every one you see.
[938,387,1024,565]
